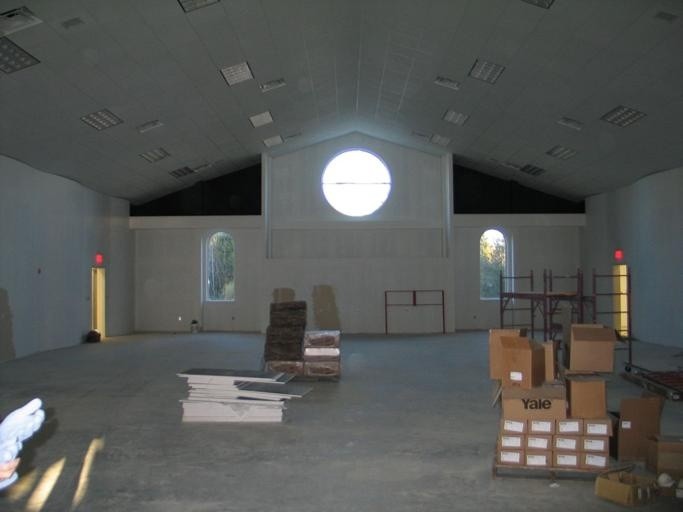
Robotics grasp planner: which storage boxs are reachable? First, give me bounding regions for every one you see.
[489,322,683,507]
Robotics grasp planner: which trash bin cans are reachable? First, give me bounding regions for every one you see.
[190,320,199,334]
